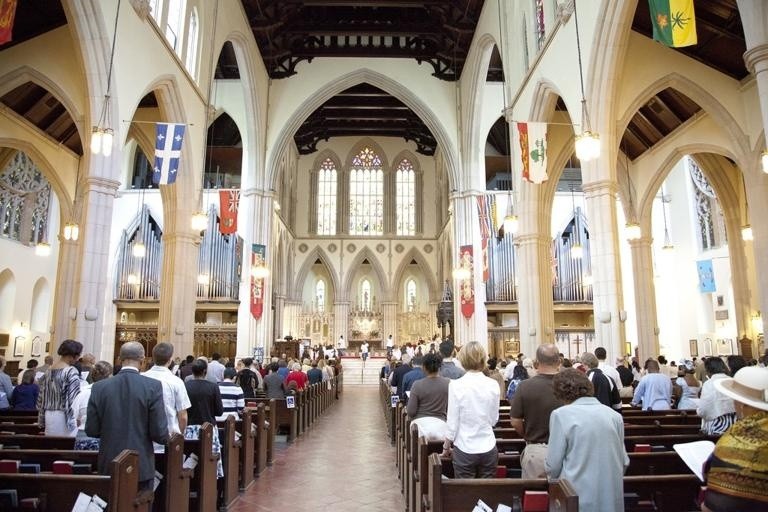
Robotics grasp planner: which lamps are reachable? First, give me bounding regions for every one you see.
[91,1,121,157]
[130,158,147,261]
[737,171,755,242]
[34,183,57,259]
[557,157,587,259]
[760,149,768,173]
[621,133,641,240]
[60,142,81,242]
[657,173,675,259]
[559,0,602,161]
[492,0,520,235]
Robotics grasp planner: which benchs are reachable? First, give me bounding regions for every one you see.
[0,371,343,512]
[379,378,723,512]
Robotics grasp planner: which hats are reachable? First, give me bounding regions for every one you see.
[715,366,768,410]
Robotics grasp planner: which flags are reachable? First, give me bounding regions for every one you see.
[476,193,498,240]
[0,2,20,45]
[217,187,242,237]
[152,120,185,189]
[647,0,699,50]
[516,120,551,185]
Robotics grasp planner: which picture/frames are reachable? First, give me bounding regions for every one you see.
[688,339,698,357]
[505,340,521,354]
[716,338,733,356]
[703,336,713,358]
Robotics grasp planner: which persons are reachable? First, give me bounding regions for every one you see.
[381,335,767,511]
[361,340,369,360]
[0,334,346,491]
